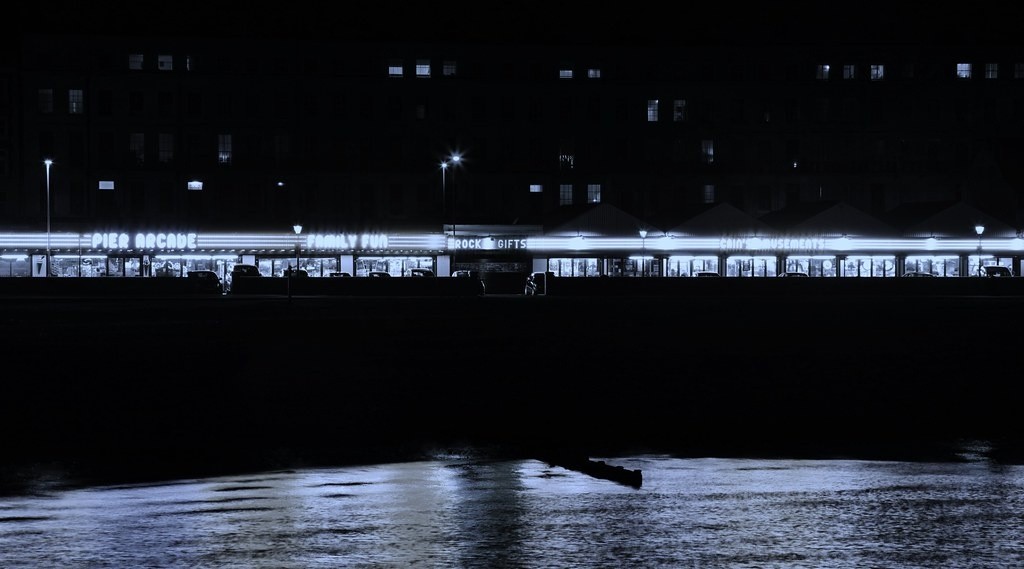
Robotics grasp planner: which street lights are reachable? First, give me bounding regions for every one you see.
[442,163,448,210]
[44,158,55,254]
